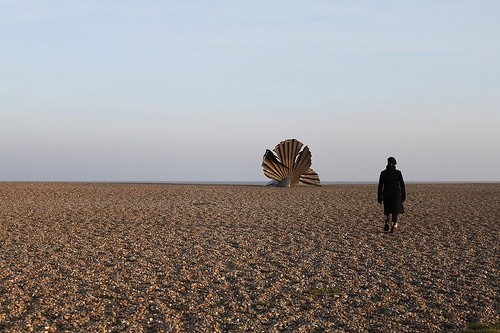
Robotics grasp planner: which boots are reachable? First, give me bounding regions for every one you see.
[384,219,390,232]
[391,222,398,234]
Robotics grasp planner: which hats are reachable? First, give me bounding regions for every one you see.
[387,157,397,164]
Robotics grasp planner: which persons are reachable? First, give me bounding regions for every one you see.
[377,156,406,234]
